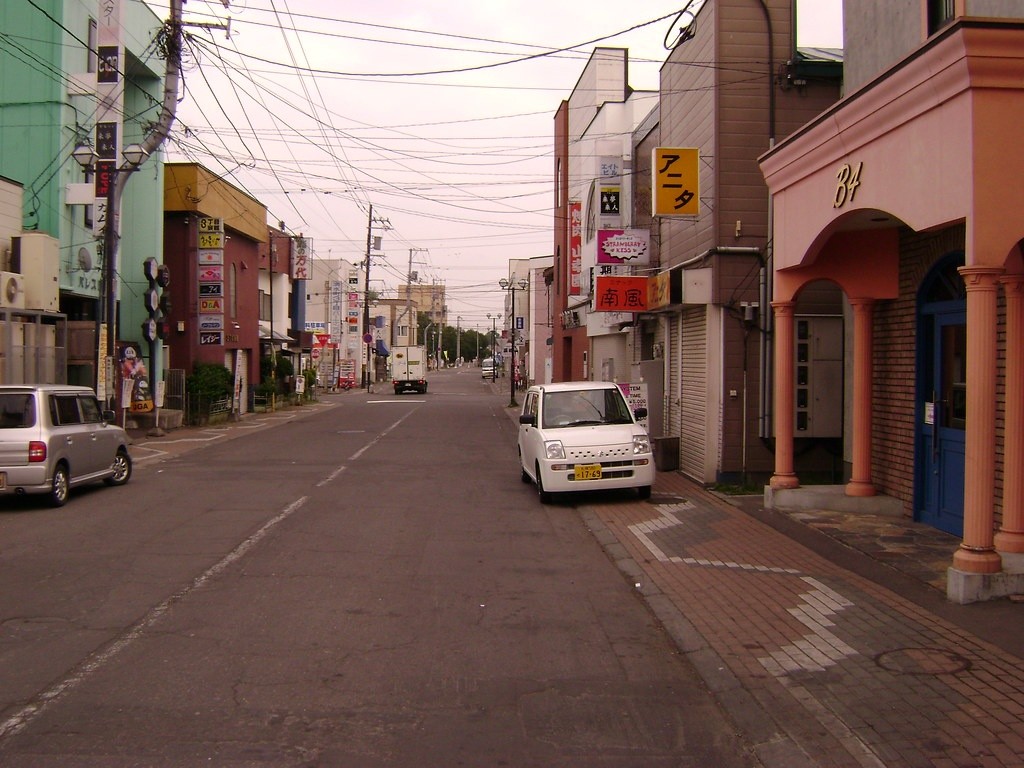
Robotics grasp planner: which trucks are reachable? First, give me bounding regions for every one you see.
[391,345,429,394]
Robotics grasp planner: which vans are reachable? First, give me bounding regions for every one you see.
[481,359,498,379]
[0,382,133,508]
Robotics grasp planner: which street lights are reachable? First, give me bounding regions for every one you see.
[72,143,151,425]
[487,313,503,381]
[498,278,530,409]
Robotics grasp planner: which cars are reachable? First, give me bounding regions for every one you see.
[518,381,657,504]
